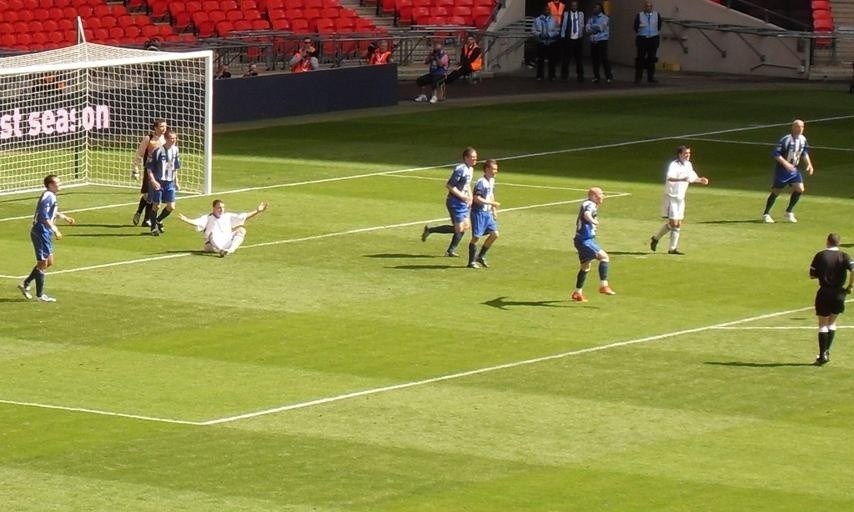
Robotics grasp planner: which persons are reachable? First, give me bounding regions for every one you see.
[548,0,565,24]
[809,233,853,366]
[456,32,471,63]
[572,187,615,302]
[447,35,483,84]
[244,63,260,78]
[33,72,64,97]
[467,160,501,269]
[146,39,165,85]
[585,4,615,85]
[422,147,477,257]
[532,6,559,82]
[415,44,449,104]
[132,120,167,226]
[560,1,586,84]
[650,145,708,254]
[147,131,181,237]
[214,63,232,80]
[291,38,320,73]
[178,200,269,256]
[763,120,814,224]
[368,40,393,65]
[18,174,75,302]
[634,2,662,86]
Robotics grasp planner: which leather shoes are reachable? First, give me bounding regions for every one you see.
[648,78,660,83]
[632,78,641,83]
[576,77,585,83]
[561,76,568,81]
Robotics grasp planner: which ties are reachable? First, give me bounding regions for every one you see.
[573,14,578,34]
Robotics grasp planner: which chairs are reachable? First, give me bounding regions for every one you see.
[811,0,834,49]
[377,0,499,43]
[0,1,392,60]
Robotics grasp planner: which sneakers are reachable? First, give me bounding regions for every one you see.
[668,249,684,255]
[18,282,32,300]
[476,256,489,267]
[537,77,542,81]
[816,351,829,359]
[572,292,587,301]
[421,225,430,242]
[142,220,152,227]
[415,95,427,102]
[430,96,438,103]
[151,225,159,236]
[36,293,56,302]
[591,78,600,82]
[467,262,480,269]
[599,286,615,295]
[219,249,229,257]
[133,211,141,225]
[815,357,827,365]
[607,78,615,83]
[783,212,797,223]
[762,215,775,224]
[445,251,459,257]
[156,217,166,232]
[550,77,556,81]
[651,235,658,251]
